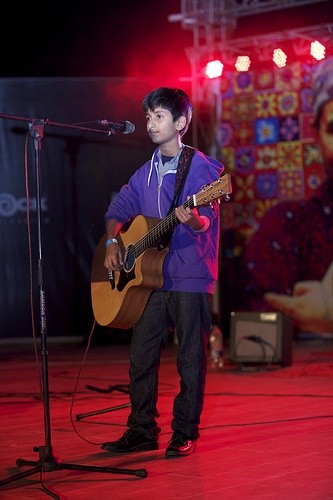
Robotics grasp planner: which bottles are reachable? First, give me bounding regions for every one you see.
[210,327,223,368]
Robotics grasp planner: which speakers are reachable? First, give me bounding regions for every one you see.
[228,312,293,367]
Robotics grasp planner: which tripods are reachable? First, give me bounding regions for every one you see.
[0,123,148,486]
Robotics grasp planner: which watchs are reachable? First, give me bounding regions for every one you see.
[104,238,118,247]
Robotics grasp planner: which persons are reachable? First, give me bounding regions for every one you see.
[264,260,333,334]
[100,87,225,457]
[239,57,333,311]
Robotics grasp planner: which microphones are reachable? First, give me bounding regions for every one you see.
[97,120,136,135]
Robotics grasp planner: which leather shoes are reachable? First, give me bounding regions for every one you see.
[101,428,159,451]
[165,439,194,455]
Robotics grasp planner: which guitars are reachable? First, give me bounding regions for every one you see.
[91,173,233,329]
